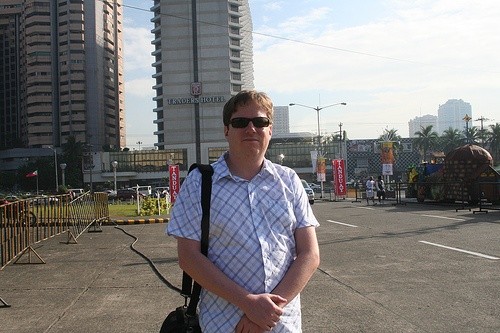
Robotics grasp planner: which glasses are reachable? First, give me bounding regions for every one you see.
[229,117,270,128]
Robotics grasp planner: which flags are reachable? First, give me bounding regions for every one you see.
[26,170,37,177]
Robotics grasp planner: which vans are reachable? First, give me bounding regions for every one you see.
[68,189,84,200]
[154,187,170,194]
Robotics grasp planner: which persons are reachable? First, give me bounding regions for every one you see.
[166,90,320,333]
[366,175,386,206]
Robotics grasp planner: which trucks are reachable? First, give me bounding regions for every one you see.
[132,186,152,195]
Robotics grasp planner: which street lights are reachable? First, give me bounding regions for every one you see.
[289,102,347,197]
[61,163,67,186]
[48,147,59,193]
[112,160,118,201]
[136,141,142,150]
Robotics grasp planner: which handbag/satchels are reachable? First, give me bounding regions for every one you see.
[159,305,202,333]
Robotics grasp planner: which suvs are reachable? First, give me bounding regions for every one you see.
[117,188,146,201]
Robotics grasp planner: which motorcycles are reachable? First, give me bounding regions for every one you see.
[0,193,37,227]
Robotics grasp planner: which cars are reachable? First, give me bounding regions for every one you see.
[300,179,314,205]
[308,183,322,191]
[354,180,379,187]
[6,193,59,204]
